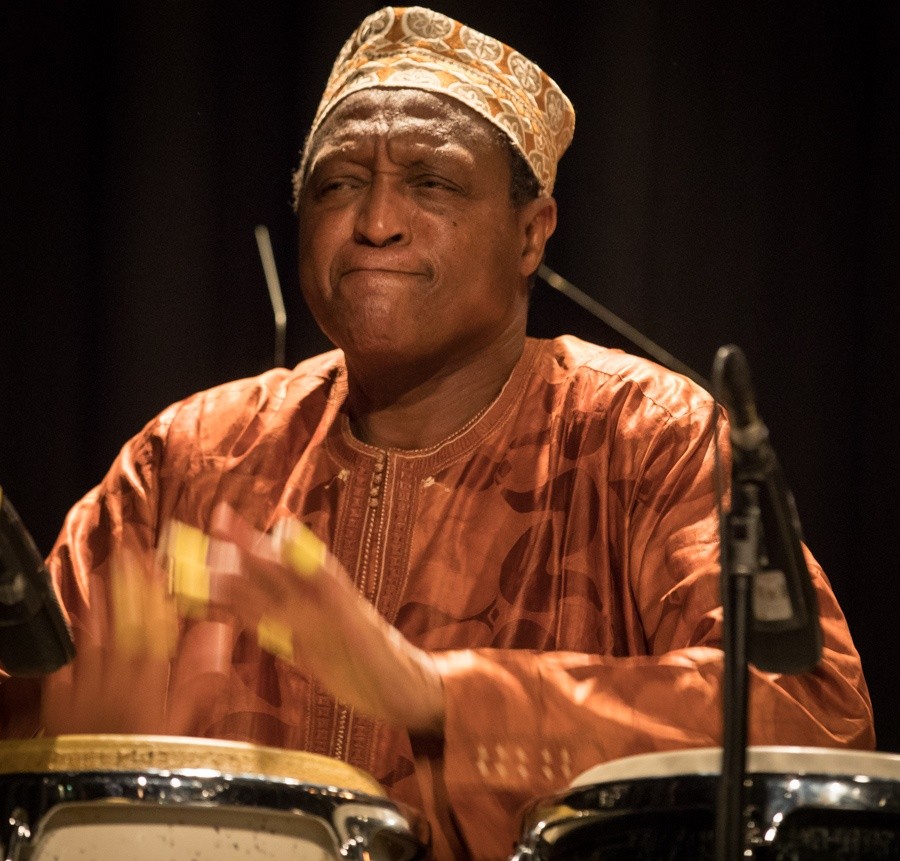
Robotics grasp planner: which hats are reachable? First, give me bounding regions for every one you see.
[310,4,579,200]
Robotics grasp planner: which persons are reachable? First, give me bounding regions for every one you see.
[1,5,880,861]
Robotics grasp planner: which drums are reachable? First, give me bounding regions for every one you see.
[2,732,398,861]
[513,737,899,861]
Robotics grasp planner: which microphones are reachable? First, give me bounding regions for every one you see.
[720,346,825,673]
